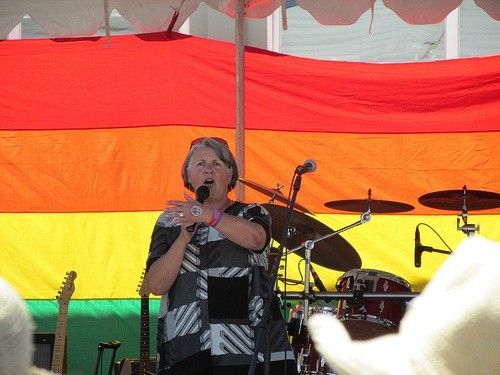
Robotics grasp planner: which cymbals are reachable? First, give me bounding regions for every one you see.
[259,203,363,271]
[323,199,416,215]
[417,189,500,211]
[238,176,316,216]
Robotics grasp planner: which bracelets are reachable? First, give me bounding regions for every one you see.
[207,208,224,228]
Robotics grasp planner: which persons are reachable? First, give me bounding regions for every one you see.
[306,236,500,375]
[144,137,272,375]
[0,278,61,375]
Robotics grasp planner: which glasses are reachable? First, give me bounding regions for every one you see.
[190,137,229,150]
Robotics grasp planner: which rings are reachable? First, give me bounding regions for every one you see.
[179,212,184,218]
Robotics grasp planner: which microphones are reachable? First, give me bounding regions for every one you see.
[186,185,210,231]
[415,226,421,266]
[296,160,316,174]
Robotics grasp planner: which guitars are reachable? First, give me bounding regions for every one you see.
[126,267,156,375]
[50,270,78,375]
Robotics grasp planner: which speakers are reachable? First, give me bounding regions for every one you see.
[31,332,67,375]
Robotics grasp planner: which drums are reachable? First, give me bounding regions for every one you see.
[286,305,340,375]
[336,268,414,340]
